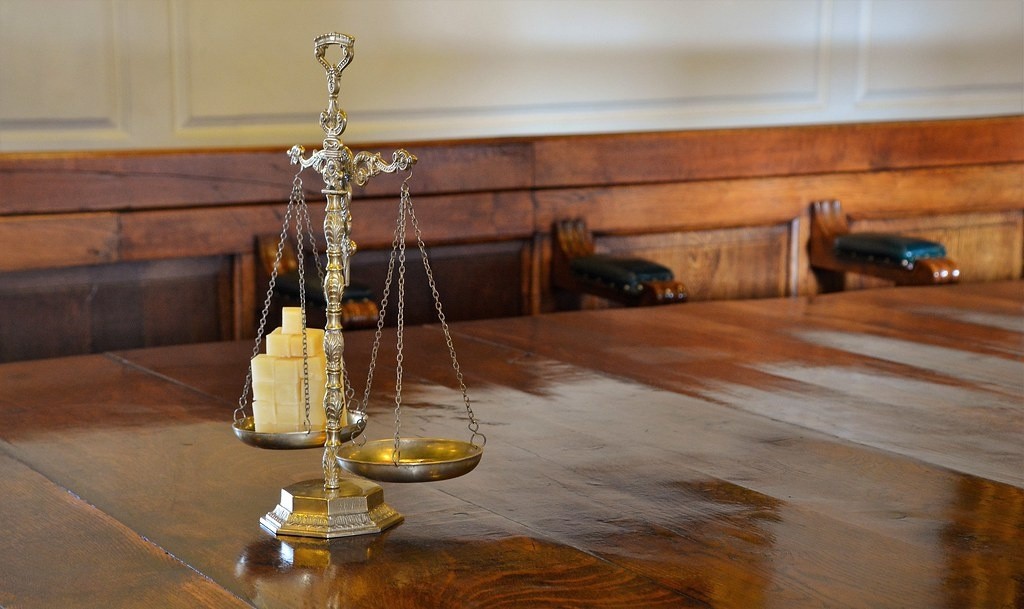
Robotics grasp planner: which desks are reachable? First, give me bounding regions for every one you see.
[1,276,1024,609]
